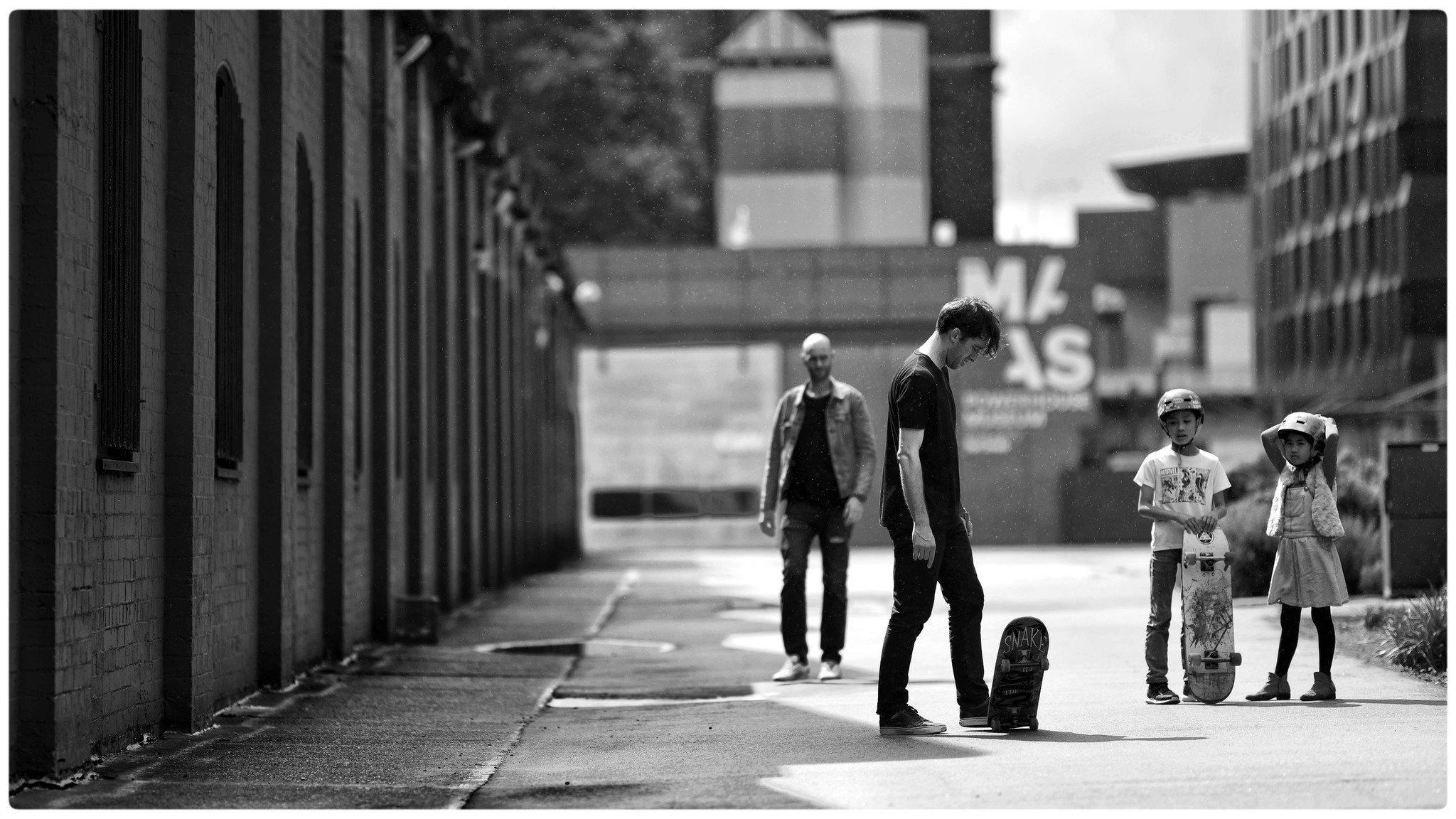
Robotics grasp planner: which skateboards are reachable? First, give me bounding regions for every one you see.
[1180,514,1243,706]
[986,615,1051,737]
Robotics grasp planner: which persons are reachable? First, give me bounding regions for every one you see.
[758,333,878,681]
[1132,388,1232,705]
[875,297,1002,736]
[1246,411,1349,702]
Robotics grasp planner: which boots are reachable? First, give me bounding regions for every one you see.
[1300,672,1336,701]
[1246,672,1290,701]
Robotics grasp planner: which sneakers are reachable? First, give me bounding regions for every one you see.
[880,706,947,734]
[1146,682,1180,705]
[1182,681,1199,702]
[772,655,810,681]
[959,696,990,726]
[818,660,842,680]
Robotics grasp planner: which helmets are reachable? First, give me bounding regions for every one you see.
[1157,388,1204,423]
[1278,412,1325,456]
